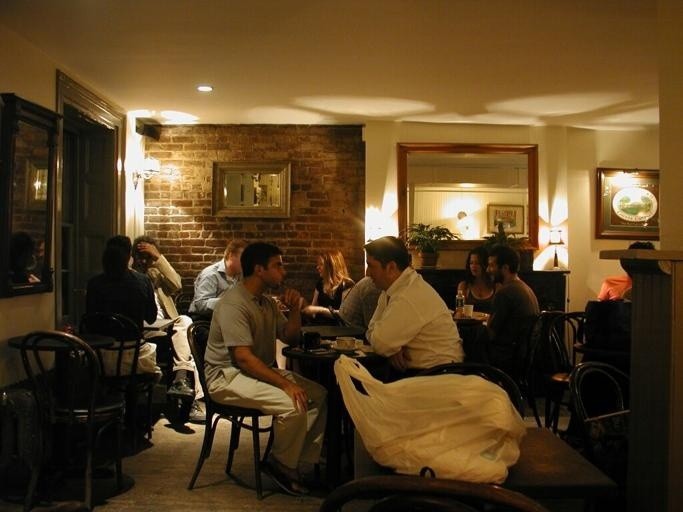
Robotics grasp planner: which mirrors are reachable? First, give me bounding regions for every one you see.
[0,92,63,298]
[397,142,539,251]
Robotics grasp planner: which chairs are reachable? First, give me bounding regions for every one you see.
[21,331,123,512]
[175,292,193,316]
[322,306,633,512]
[79,312,157,449]
[187,321,276,499]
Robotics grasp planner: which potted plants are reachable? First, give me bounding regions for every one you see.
[400,223,463,266]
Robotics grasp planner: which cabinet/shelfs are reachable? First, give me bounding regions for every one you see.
[413,270,570,342]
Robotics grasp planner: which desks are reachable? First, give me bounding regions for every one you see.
[282,324,368,346]
[9,331,115,399]
[281,340,375,489]
[142,318,174,423]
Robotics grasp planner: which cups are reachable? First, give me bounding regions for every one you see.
[461,304,473,319]
[336,337,355,350]
[301,330,320,353]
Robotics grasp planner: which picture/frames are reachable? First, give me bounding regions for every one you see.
[487,204,526,235]
[213,161,291,219]
[595,167,660,241]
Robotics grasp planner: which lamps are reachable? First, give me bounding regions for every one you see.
[548,230,565,270]
[132,156,178,190]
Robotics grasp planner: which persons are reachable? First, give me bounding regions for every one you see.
[83,234,162,378]
[596,238,656,303]
[338,273,382,329]
[300,245,356,324]
[475,243,541,384]
[202,241,333,496]
[28,231,44,266]
[131,236,209,427]
[7,231,43,288]
[187,238,286,356]
[308,235,471,497]
[457,246,501,314]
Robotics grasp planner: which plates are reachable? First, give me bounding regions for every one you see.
[473,311,491,321]
[329,339,364,349]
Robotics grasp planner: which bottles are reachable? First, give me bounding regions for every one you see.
[454,288,464,319]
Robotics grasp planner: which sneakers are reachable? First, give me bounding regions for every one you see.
[167,377,195,399]
[259,453,310,496]
[181,399,207,422]
